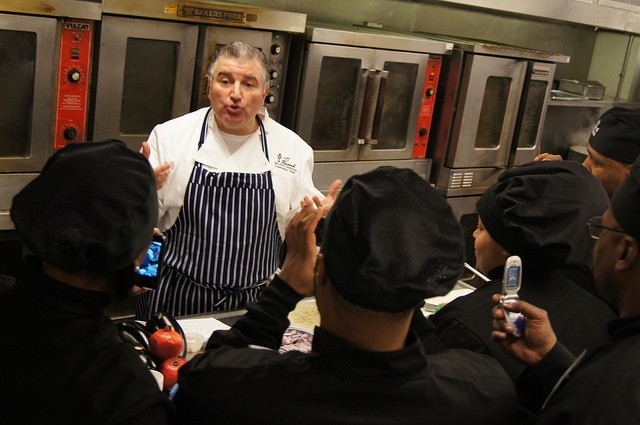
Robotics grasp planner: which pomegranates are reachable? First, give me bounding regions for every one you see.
[162,357,188,386]
[151,326,183,357]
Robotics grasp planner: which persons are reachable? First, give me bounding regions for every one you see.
[490,155,639,423]
[0,138,173,424]
[137,40,343,318]
[428,159,617,419]
[174,164,521,425]
[533,106,640,196]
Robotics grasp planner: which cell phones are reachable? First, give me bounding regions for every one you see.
[134,233,166,290]
[500,256,525,337]
[315,214,328,247]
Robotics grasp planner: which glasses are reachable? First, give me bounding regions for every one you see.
[584,216,626,240]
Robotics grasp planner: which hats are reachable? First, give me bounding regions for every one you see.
[588,107,639,165]
[321,165,465,310]
[10,138,159,274]
[611,163,640,238]
[475,160,611,278]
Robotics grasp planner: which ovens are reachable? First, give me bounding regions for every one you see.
[281,22,455,192]
[91,0,308,157]
[413,30,572,199]
[0,0,103,232]
